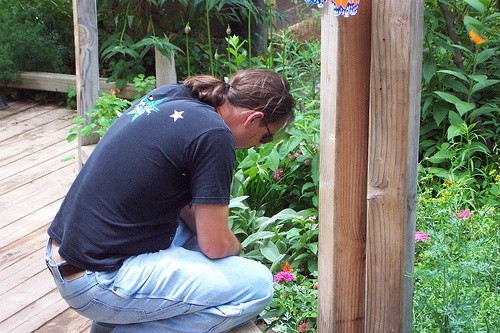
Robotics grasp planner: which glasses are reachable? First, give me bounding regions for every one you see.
[260,115,274,145]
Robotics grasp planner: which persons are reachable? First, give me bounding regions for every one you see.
[45,67,301,333]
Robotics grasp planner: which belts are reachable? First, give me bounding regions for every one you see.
[43,238,86,282]
